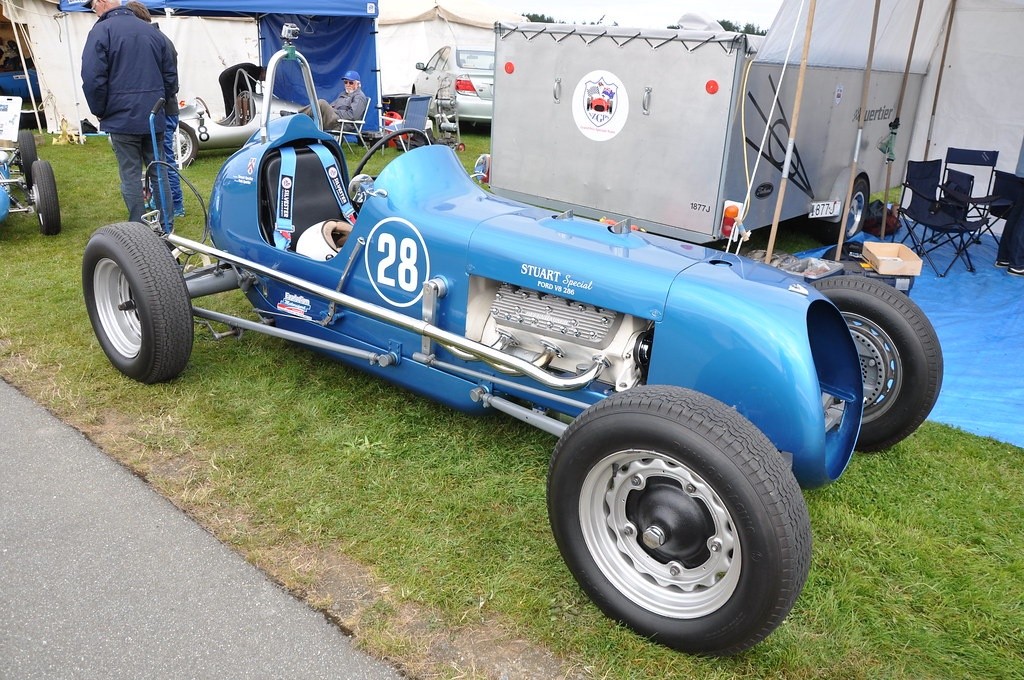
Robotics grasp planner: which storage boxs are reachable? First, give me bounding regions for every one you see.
[835,261,915,297]
[862,241,923,276]
[781,257,844,284]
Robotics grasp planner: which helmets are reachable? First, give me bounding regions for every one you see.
[296,219,352,261]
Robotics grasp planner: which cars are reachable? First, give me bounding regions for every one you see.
[82,22,944,655]
[411,43,495,130]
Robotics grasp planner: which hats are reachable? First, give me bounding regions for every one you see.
[82,0,92,9]
[341,71,360,81]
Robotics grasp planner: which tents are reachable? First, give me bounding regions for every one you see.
[724,0,1024,265]
[0,0,530,136]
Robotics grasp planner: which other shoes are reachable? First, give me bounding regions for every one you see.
[1007,267,1024,276]
[145,199,156,210]
[995,261,1009,268]
[174,208,186,217]
[280,110,292,116]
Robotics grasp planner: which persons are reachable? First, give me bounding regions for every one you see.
[279,70,367,135]
[79,0,177,240]
[120,1,185,219]
[993,133,1024,277]
[0,36,26,72]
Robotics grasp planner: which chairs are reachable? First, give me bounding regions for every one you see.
[379,96,433,157]
[892,147,1024,278]
[218,69,254,127]
[261,146,358,254]
[324,97,371,159]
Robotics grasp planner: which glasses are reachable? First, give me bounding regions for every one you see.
[91,0,98,12]
[344,81,353,85]
